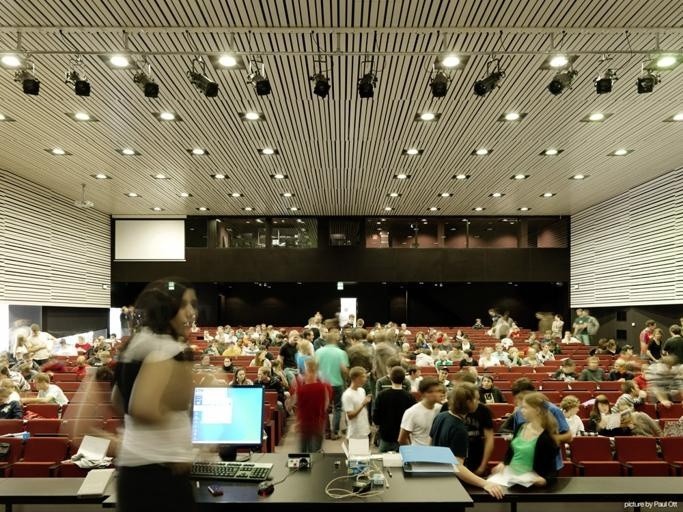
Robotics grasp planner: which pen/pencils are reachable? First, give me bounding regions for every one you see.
[386,468,392,477]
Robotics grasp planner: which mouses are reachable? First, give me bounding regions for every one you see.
[258,481,274,497]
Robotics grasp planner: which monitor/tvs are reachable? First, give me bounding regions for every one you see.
[191,385,265,461]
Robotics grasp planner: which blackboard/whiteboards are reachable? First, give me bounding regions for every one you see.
[340,297,356,327]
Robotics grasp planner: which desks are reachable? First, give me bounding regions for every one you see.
[103,453,474,512]
[0,477,118,512]
[458,477,683,512]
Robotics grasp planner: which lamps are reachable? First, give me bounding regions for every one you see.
[0,32,683,98]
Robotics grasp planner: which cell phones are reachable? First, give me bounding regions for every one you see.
[208,485,224,495]
[352,479,371,489]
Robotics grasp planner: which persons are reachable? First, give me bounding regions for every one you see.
[0,277,683,512]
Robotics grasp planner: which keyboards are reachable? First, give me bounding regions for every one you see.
[190,461,275,480]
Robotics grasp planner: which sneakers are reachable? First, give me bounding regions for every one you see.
[323,430,341,441]
